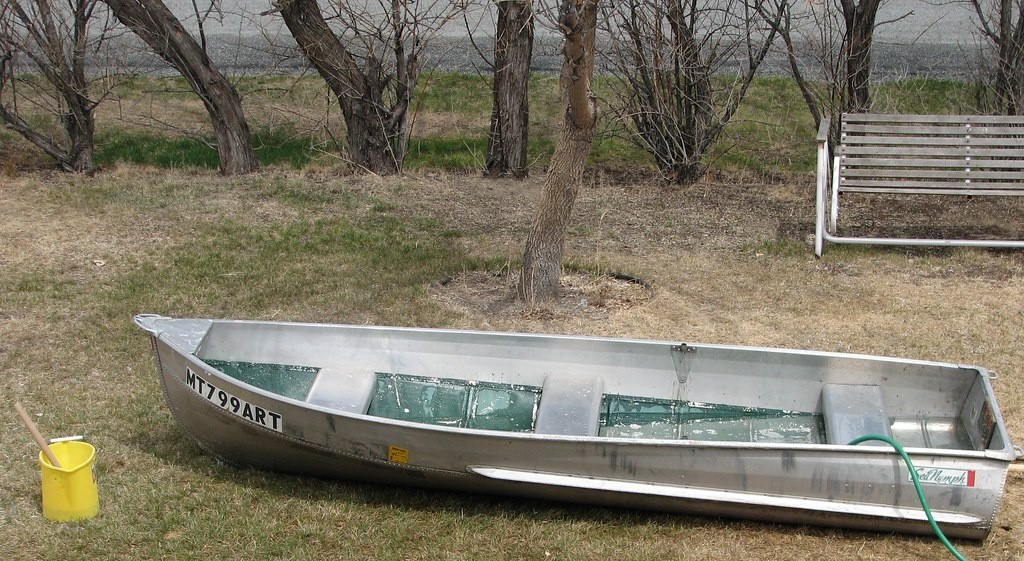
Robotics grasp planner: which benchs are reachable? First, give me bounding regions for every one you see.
[815,112,1024,259]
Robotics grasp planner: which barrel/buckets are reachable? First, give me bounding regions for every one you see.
[37,435,100,522]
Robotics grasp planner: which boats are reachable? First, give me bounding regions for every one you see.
[133,311,1013,542]
